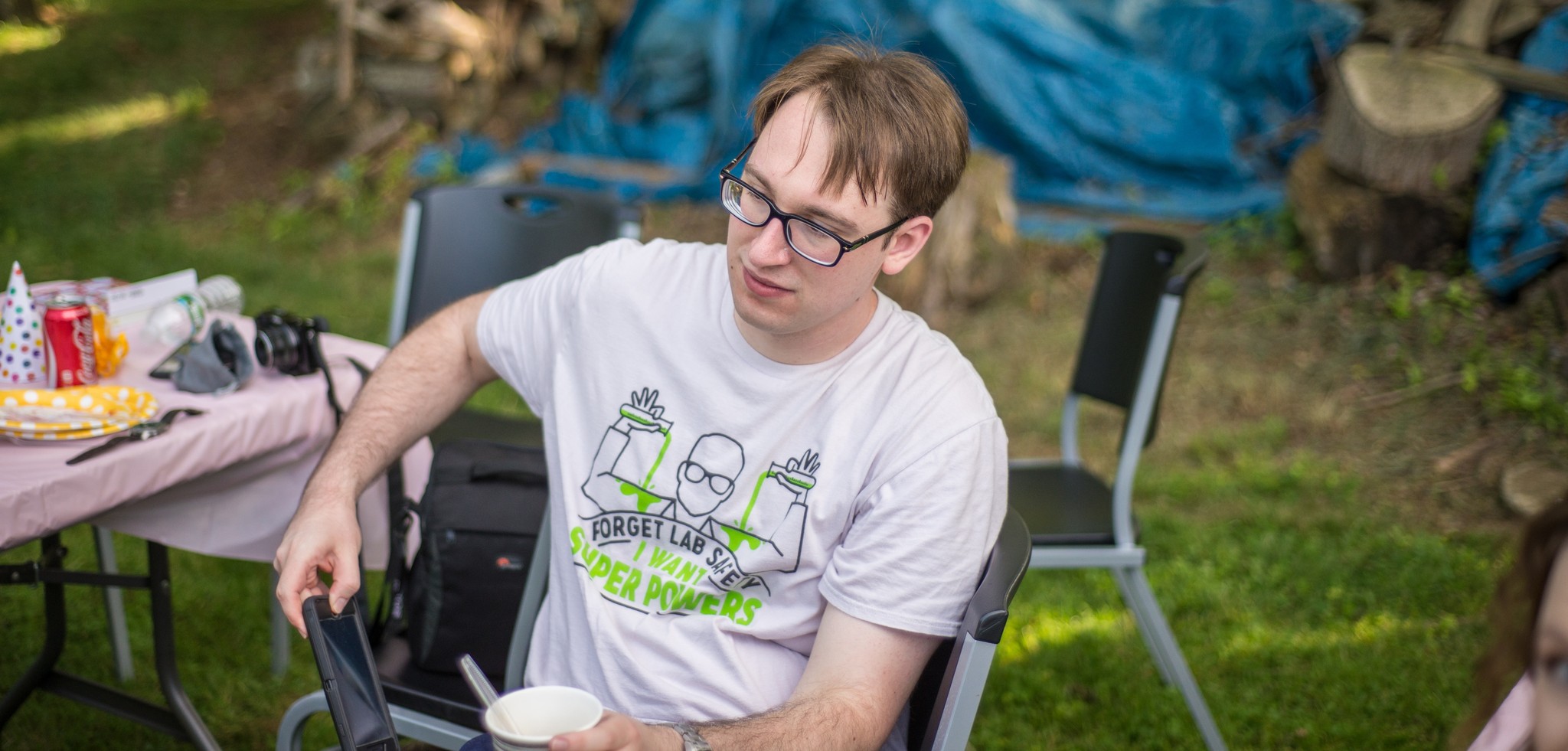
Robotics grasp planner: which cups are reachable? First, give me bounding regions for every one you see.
[483,685,603,751]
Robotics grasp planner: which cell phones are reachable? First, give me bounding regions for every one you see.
[303,593,400,751]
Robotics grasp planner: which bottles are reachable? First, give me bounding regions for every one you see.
[140,273,245,345]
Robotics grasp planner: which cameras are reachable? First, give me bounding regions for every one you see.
[253,307,323,376]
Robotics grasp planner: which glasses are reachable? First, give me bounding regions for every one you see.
[720,134,907,268]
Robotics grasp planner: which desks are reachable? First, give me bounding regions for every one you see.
[0,278,433,751]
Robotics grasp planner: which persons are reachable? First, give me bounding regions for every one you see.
[1443,491,1568,751]
[272,12,1010,751]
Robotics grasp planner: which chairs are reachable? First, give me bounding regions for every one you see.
[1009,229,1229,751]
[278,181,1033,750]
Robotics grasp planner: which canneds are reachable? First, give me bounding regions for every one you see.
[41,294,97,391]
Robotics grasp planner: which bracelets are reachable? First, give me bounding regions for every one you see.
[657,722,712,751]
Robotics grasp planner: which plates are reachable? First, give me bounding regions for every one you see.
[0,386,159,447]
